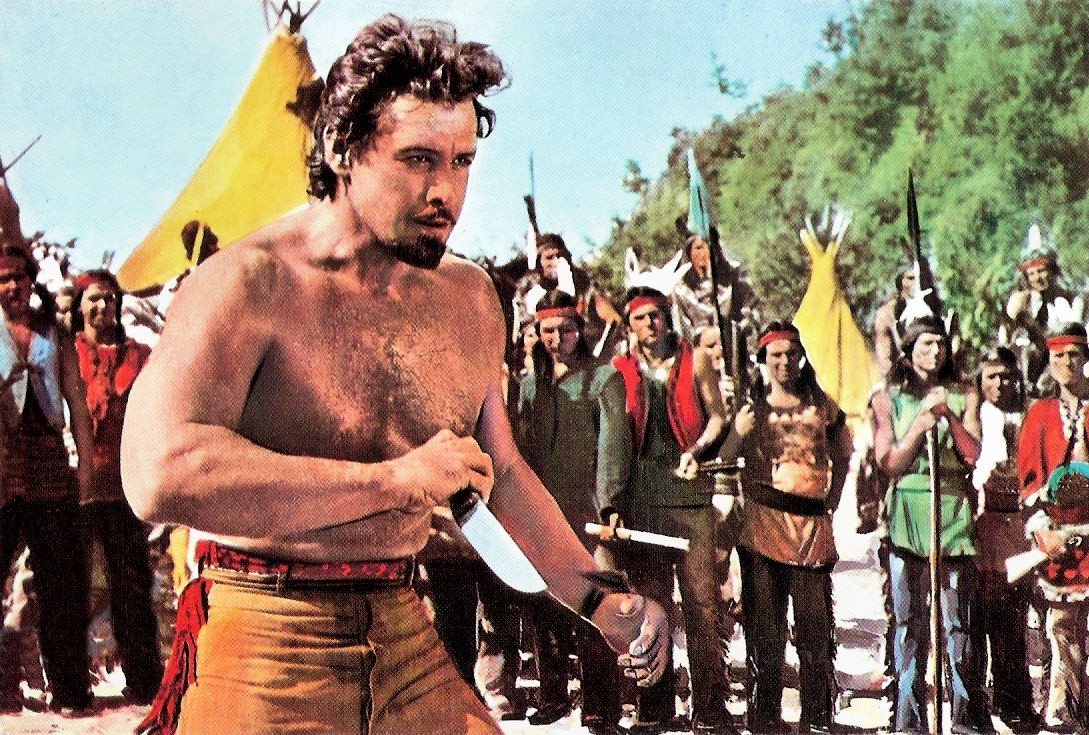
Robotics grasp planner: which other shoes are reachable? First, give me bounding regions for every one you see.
[487,667,1088,734]
[5,653,155,714]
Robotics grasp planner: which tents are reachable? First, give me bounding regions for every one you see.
[118,0,329,295]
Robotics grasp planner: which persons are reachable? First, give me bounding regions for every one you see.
[0,163,1089,735]
[119,12,668,734]
